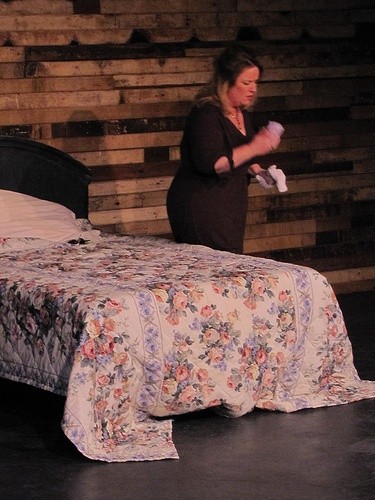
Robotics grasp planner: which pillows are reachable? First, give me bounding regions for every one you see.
[0,190,80,240]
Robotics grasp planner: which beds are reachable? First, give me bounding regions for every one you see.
[0,133,375,462]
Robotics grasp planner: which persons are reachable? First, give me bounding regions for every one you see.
[166,46,279,259]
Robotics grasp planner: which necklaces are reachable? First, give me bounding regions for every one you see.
[226,106,243,133]
